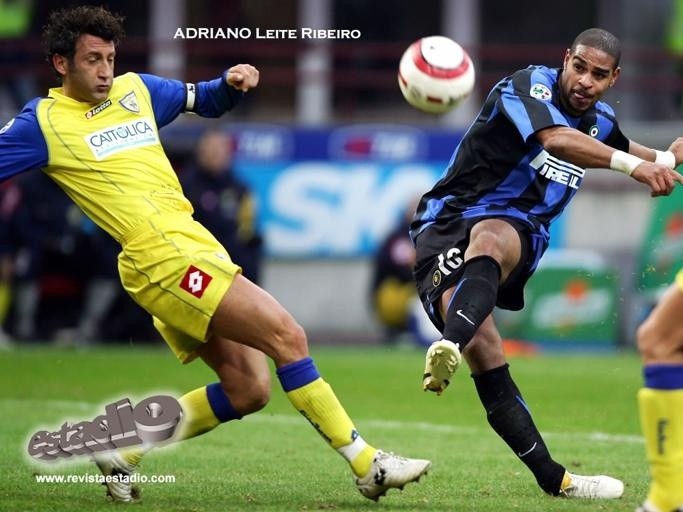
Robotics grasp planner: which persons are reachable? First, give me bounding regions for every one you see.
[0,2,430,505]
[630,267,683,512]
[181,125,259,286]
[0,166,162,344]
[369,198,445,347]
[407,28,682,501]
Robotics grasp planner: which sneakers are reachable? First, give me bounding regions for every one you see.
[93,450,134,502]
[423,339,460,396]
[355,449,432,502]
[559,470,624,498]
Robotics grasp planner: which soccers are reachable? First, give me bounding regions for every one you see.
[396,35,475,115]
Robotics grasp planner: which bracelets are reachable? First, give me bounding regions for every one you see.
[610,150,646,176]
[652,149,675,170]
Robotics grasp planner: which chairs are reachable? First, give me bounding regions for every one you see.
[215,121,294,161]
[157,121,217,157]
[323,123,432,165]
